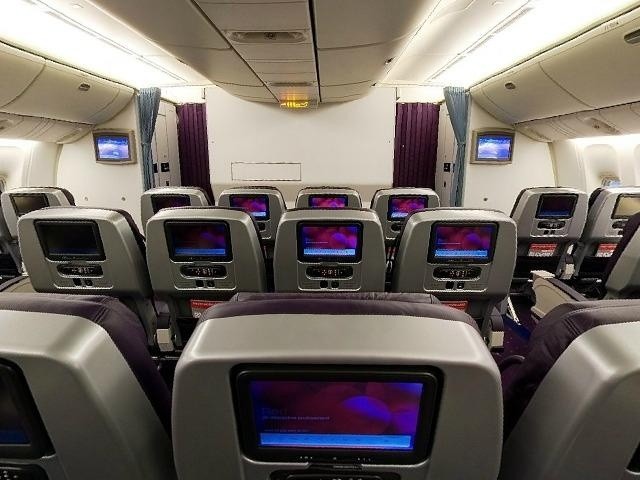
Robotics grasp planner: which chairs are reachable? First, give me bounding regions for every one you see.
[0,186,76,239]
[273,208,387,292]
[295,187,362,208]
[510,186,640,480]
[140,186,213,236]
[391,207,518,351]
[0,190,21,275]
[172,292,504,480]
[155,206,267,344]
[218,186,287,258]
[1,292,172,479]
[17,206,156,346]
[369,186,440,284]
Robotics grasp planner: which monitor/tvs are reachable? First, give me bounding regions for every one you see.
[476,134,512,162]
[10,194,47,215]
[35,220,105,262]
[152,194,190,213]
[388,196,428,221]
[536,195,577,219]
[229,195,269,222]
[612,194,640,218]
[234,366,442,464]
[0,355,48,459]
[427,221,498,265]
[309,195,348,208]
[164,221,232,263]
[296,220,362,263]
[95,133,130,162]
